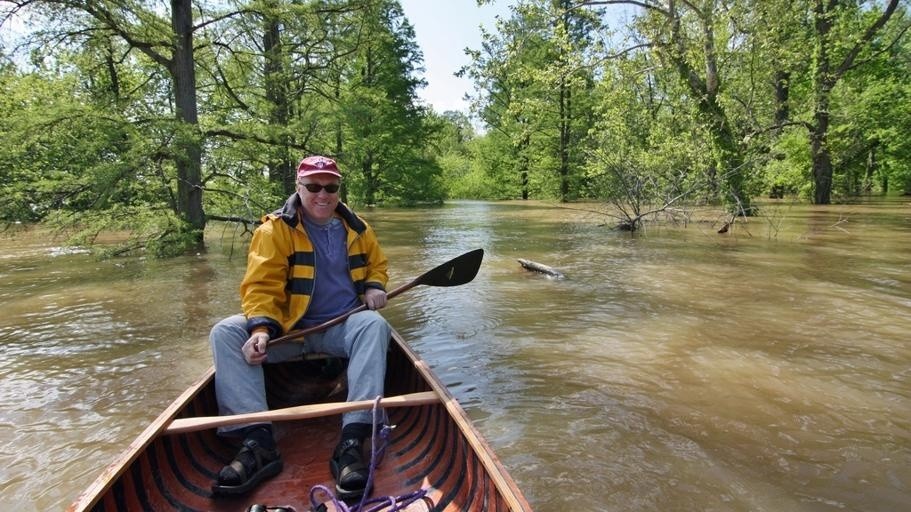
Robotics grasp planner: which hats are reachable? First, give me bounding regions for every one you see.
[297,156,341,177]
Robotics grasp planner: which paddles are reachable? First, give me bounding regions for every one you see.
[255,248,484,348]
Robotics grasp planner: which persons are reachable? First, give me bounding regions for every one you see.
[211,155,393,498]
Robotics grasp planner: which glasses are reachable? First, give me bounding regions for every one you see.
[299,181,341,193]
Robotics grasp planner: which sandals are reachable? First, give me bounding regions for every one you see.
[329,438,373,498]
[213,435,284,496]
